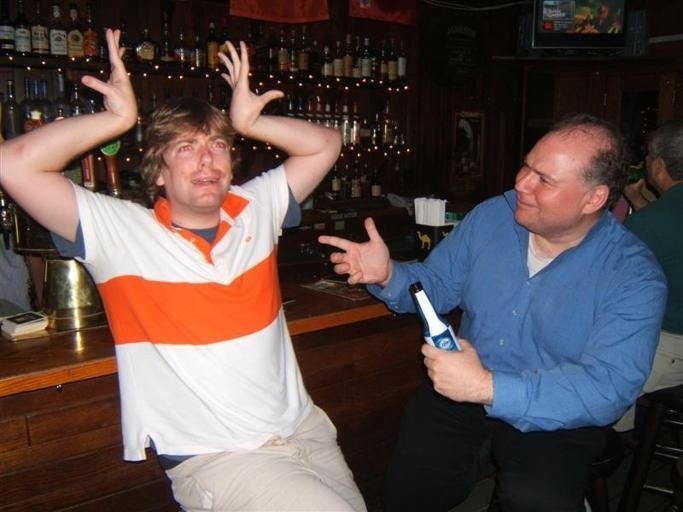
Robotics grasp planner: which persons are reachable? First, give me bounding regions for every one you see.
[0,27,367,511]
[316,112,668,512]
[0,223,34,314]
[610,120,682,450]
[579,5,611,33]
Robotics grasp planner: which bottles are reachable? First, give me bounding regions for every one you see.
[410,281,460,353]
[0,1,409,202]
[624,166,646,186]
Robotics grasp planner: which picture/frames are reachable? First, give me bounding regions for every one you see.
[447,108,486,181]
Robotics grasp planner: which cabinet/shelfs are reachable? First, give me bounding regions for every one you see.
[0,1,426,283]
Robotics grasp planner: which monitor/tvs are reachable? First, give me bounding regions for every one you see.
[517,0,649,61]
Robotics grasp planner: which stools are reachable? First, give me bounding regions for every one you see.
[619,388,683,512]
